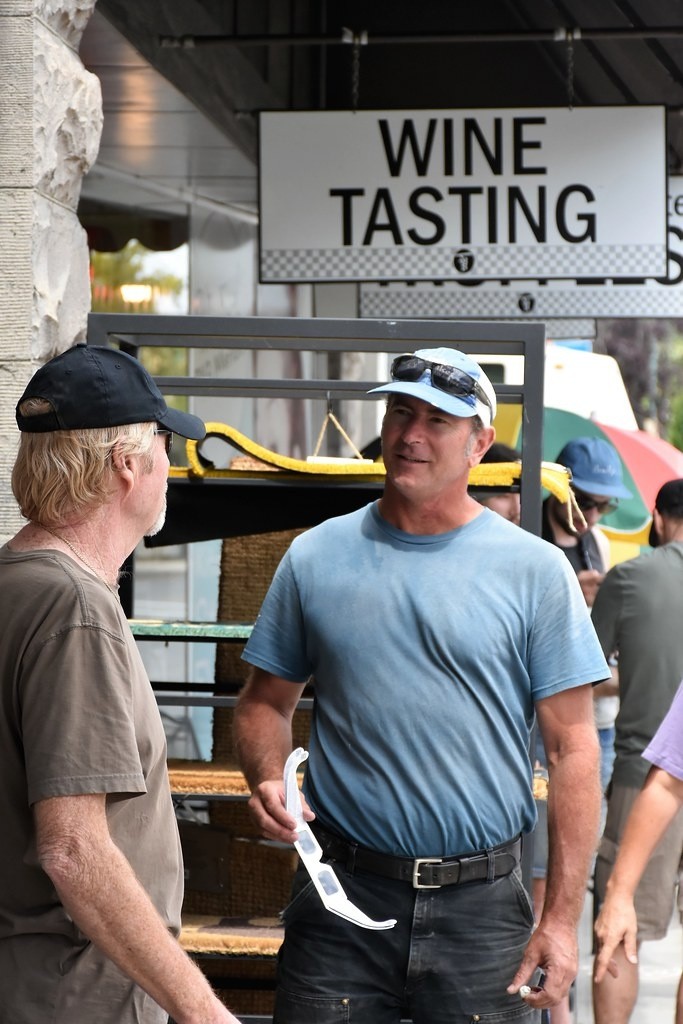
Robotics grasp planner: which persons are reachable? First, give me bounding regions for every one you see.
[589,478,683,1024]
[0,342,242,1024]
[593,679,683,984]
[230,345,602,1024]
[466,443,522,527]
[531,437,632,932]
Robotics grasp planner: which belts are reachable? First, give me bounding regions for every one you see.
[306,822,521,889]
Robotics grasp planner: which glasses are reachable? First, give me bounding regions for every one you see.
[283,747,397,930]
[574,494,619,514]
[390,356,493,423]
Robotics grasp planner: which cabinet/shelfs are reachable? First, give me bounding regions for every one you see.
[86,311,546,1024]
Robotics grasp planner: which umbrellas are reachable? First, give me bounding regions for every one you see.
[373,402,683,548]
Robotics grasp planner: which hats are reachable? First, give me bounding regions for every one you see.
[16,343,206,440]
[365,347,496,428]
[557,437,633,498]
